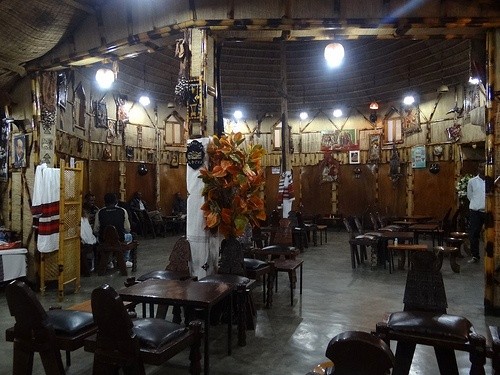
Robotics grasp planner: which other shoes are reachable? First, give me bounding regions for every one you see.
[467,256,480,263]
[107,261,114,268]
[126,261,134,267]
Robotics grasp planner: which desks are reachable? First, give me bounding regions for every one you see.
[408,224,440,246]
[268,260,304,307]
[317,225,328,245]
[117,279,233,375]
[377,232,414,269]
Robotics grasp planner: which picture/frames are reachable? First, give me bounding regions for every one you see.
[14,134,27,168]
[349,151,359,164]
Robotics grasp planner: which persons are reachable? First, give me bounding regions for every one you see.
[173,193,187,214]
[128,192,161,239]
[80,208,96,276]
[466,169,486,263]
[15,139,24,162]
[82,190,100,225]
[93,193,133,268]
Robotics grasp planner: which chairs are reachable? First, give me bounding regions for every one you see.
[6,207,500,375]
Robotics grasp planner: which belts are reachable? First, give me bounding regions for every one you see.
[474,209,484,212]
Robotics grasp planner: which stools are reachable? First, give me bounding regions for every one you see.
[64,299,92,366]
